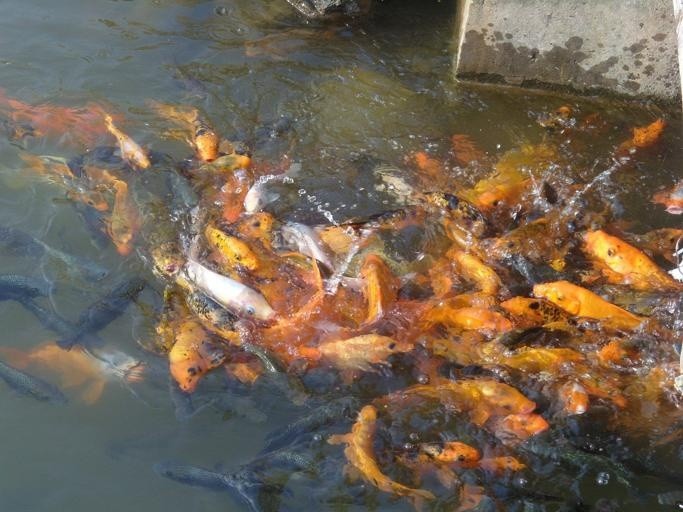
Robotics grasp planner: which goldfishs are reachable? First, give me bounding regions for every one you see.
[1,89,682,511]
